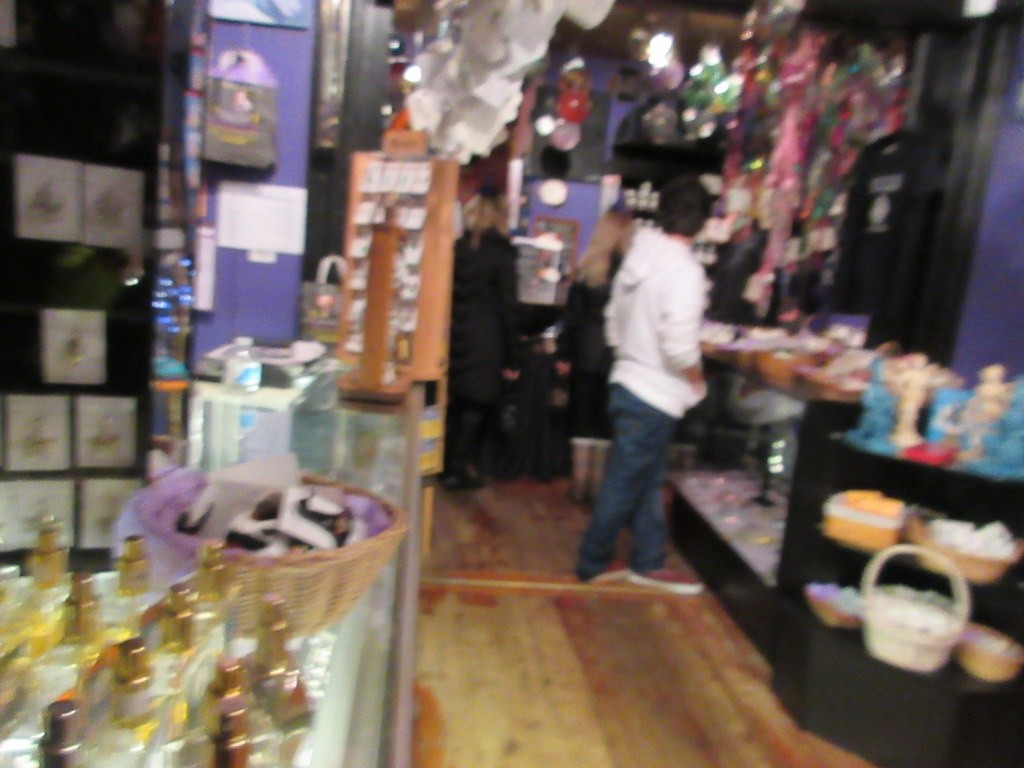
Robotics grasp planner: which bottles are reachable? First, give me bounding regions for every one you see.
[220,337,262,393]
[0,518,309,768]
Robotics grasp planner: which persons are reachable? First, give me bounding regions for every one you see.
[441,188,754,491]
[576,182,710,595]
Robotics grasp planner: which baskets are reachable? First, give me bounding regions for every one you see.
[805,583,860,629]
[908,511,1024,583]
[956,623,1024,680]
[862,544,968,672]
[151,468,406,638]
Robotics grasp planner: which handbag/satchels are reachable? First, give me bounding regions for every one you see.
[299,255,348,343]
[201,50,277,169]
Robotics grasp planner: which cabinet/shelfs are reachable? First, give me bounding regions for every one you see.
[567,281,1024,767]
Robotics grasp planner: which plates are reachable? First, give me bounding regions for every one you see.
[205,341,329,365]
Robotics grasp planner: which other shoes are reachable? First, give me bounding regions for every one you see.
[591,557,627,583]
[628,566,703,594]
